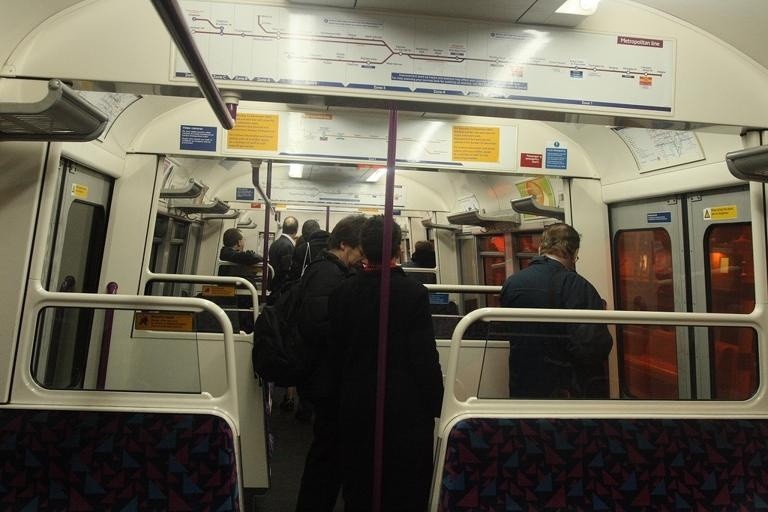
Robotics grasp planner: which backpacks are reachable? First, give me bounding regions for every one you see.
[252,257,347,387]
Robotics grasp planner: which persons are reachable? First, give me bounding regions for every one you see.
[499,223,614,399]
[220,217,445,511]
